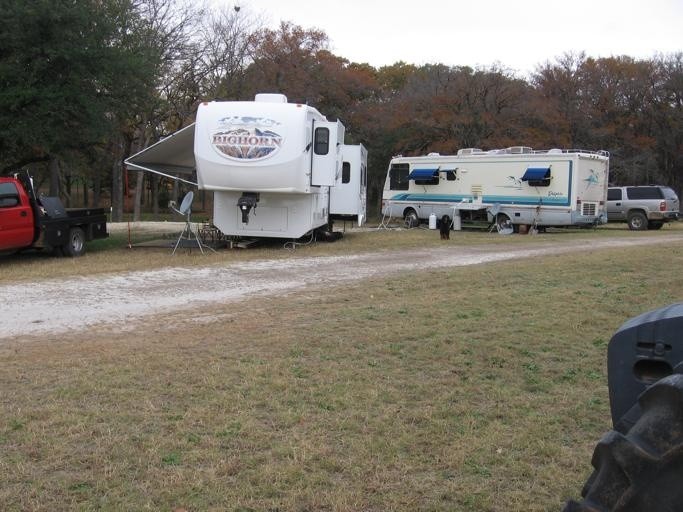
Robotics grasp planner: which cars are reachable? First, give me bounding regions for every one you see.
[606,186,680,229]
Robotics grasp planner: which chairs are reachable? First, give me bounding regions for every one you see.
[201,218,215,243]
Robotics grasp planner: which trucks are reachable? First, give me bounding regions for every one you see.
[0,170,110,257]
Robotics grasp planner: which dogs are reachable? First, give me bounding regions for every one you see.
[439,214,450,240]
